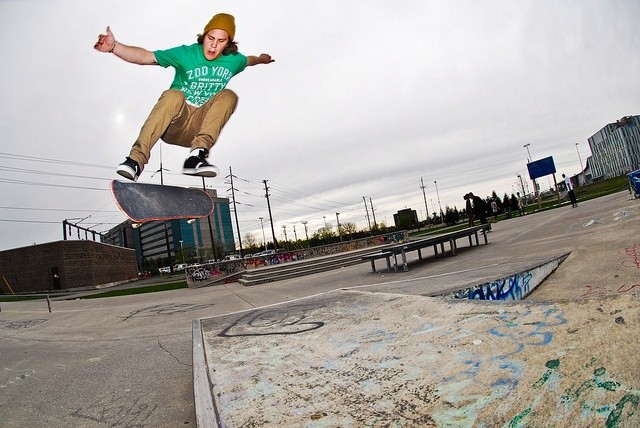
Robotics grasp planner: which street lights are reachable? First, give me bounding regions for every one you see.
[517,175,526,199]
[259,217,265,247]
[336,212,339,230]
[524,143,532,162]
[294,225,297,242]
[575,143,585,181]
[303,221,308,240]
[283,225,288,242]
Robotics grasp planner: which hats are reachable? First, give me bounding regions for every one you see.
[203,13,236,41]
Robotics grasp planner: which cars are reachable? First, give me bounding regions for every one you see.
[158,259,221,272]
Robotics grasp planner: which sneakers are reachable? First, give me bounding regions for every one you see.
[117,157,143,183]
[182,148,219,178]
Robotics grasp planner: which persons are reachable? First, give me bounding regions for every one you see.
[463,193,476,226]
[92,11,276,183]
[491,197,501,220]
[562,173,578,207]
[468,192,491,232]
[516,192,525,216]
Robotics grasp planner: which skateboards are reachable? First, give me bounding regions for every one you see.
[110,179,215,229]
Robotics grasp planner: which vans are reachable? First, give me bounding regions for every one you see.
[222,255,241,261]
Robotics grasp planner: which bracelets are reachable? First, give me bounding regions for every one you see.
[109,41,117,52]
